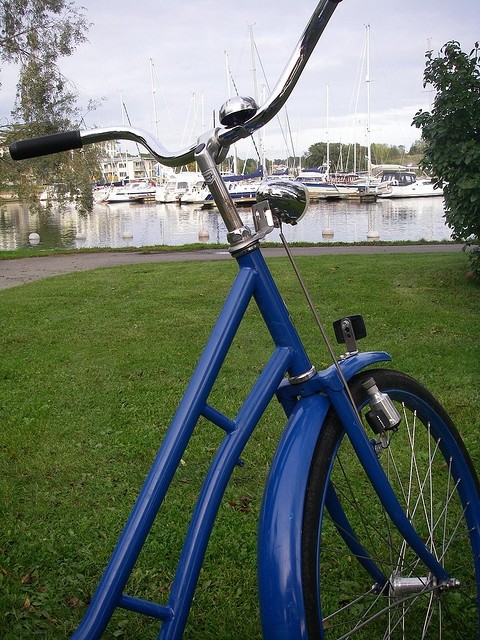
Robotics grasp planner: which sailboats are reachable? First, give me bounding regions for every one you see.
[155,85,206,203]
[248,22,382,201]
[40,13,155,210]
[377,34,454,200]
[177,47,267,207]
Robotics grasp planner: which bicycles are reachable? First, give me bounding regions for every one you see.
[6,0,479,639]
